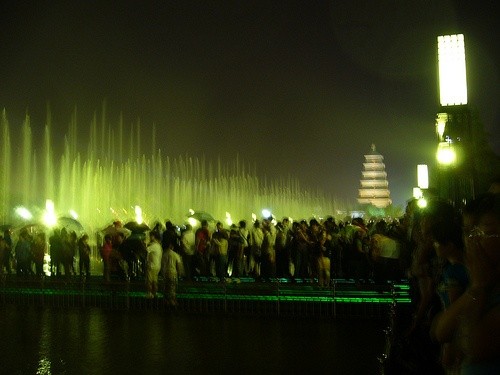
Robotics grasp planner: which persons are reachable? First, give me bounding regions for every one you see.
[1,197,500,375]
